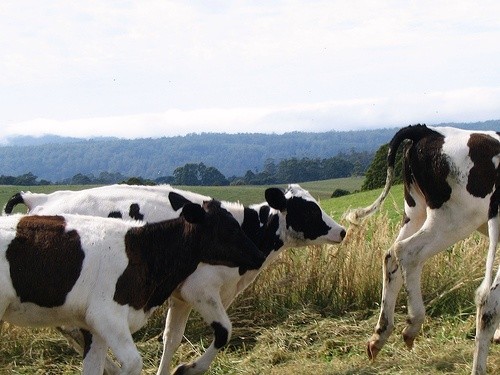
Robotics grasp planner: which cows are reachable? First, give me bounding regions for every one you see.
[346,125,500,374]
[0,183,346,375]
